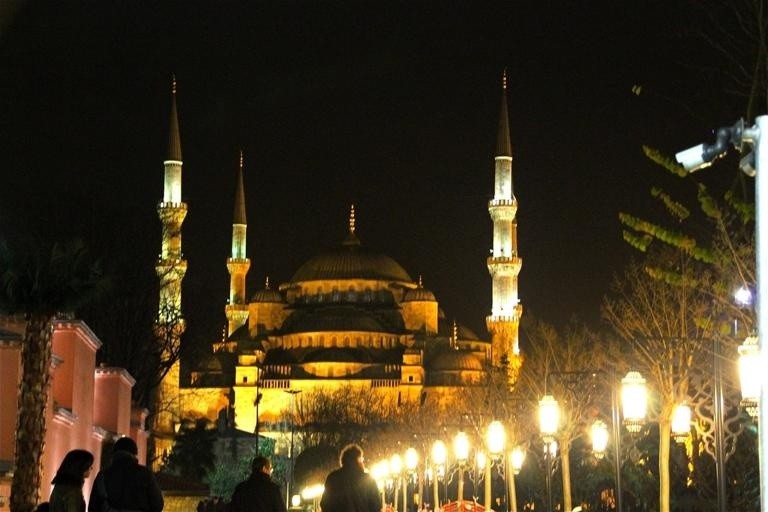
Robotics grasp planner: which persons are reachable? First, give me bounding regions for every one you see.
[49,449,94,512]
[88,437,165,512]
[229,456,288,512]
[320,444,383,512]
[197,496,226,512]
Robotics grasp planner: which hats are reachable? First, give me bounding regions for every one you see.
[111,438,138,455]
